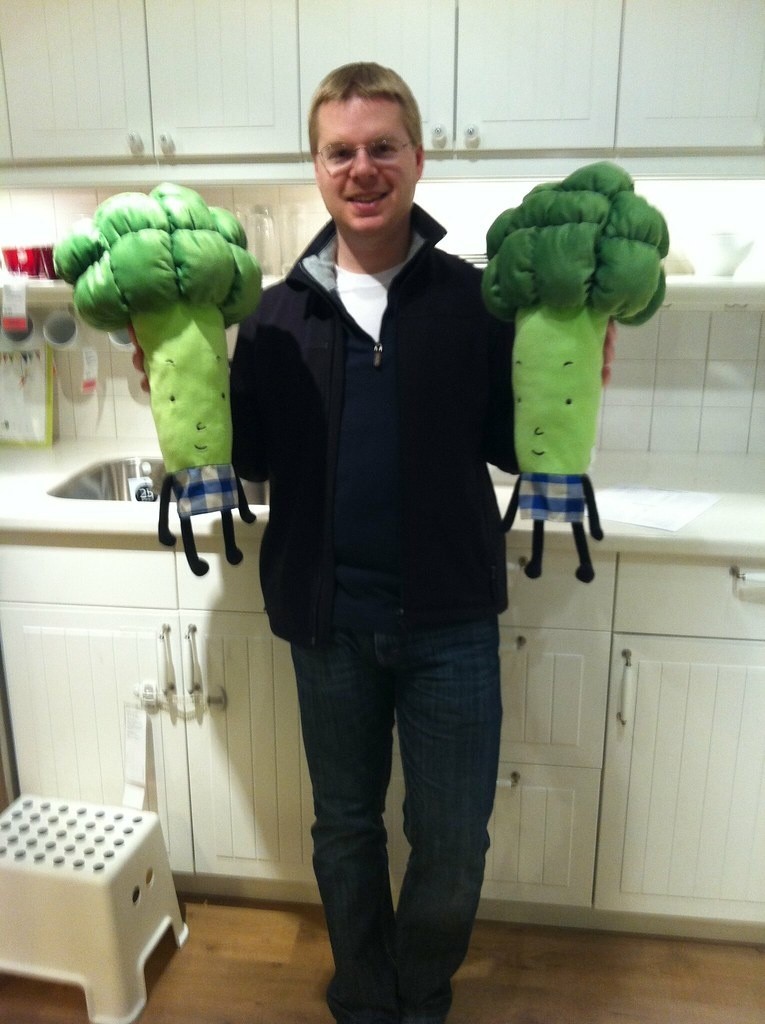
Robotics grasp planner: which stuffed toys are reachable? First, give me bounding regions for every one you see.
[479,161,669,583]
[53,182,263,577]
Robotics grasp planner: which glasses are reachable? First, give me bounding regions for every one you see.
[315,133,415,178]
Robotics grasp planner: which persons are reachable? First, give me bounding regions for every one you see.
[133,62,616,1024]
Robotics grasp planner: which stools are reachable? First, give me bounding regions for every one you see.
[1,794,190,1023]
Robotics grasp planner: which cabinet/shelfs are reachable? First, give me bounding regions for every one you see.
[594,540,765,944]
[298,0,626,184]
[2,0,303,185]
[0,534,303,905]
[616,1,765,177]
[300,535,619,929]
